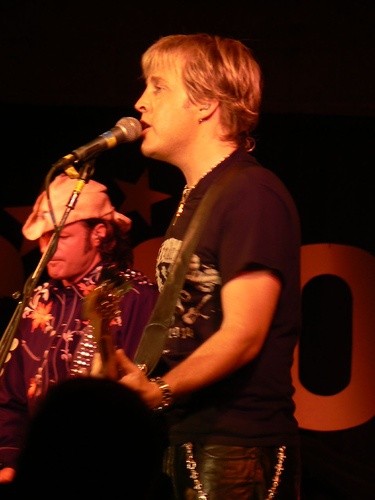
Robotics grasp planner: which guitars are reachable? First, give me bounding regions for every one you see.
[69,293,117,379]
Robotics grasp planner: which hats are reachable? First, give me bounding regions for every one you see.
[22,173,131,240]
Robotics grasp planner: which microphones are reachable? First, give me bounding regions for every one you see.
[52,117,142,173]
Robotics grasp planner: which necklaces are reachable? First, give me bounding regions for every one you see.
[172,154,230,227]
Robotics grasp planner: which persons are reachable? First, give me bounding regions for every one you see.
[0,172,159,483]
[87,33,299,500]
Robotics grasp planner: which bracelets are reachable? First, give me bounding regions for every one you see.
[150,376,172,410]
